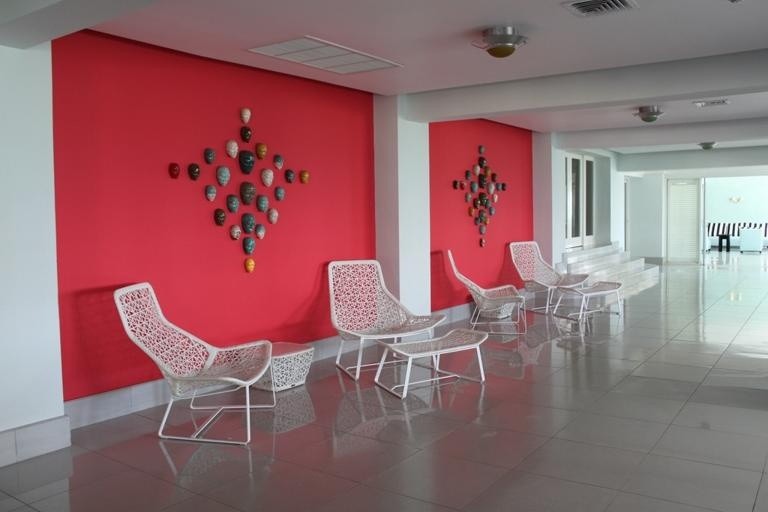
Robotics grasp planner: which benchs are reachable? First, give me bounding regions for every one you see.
[373,384,487,444]
[374,327,488,399]
[552,281,623,321]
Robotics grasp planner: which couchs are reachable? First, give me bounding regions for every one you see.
[707,222,768,249]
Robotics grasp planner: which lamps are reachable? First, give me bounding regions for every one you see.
[697,141,715,150]
[632,105,664,123]
[471,24,532,58]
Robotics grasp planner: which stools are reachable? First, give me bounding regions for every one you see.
[239,341,315,392]
[477,303,516,319]
[716,234,730,252]
[476,315,519,345]
[241,384,316,433]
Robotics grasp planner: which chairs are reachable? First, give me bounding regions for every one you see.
[520,309,585,349]
[113,281,276,445]
[509,240,590,315]
[327,259,448,381]
[447,249,528,335]
[456,334,529,394]
[739,229,764,254]
[158,439,274,512]
[332,366,434,459]
